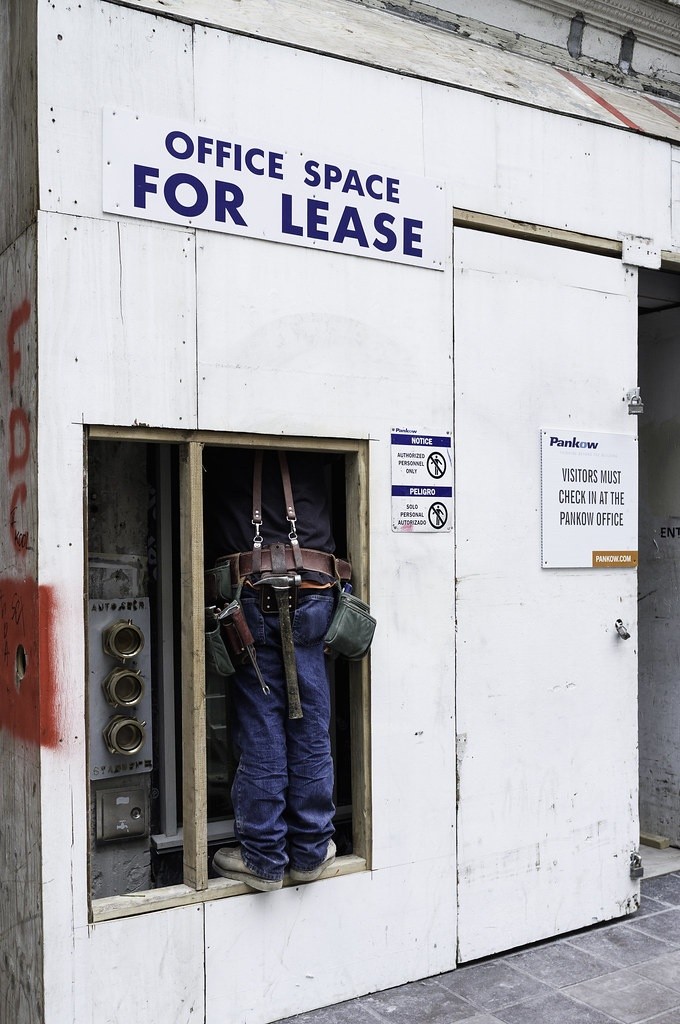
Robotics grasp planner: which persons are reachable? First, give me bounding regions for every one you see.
[207,448,339,893]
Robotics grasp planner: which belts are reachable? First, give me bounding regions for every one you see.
[214,547,351,581]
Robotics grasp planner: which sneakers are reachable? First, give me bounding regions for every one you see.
[290,838,336,881]
[213,846,282,892]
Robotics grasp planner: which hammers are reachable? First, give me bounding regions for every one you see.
[253,571,305,720]
